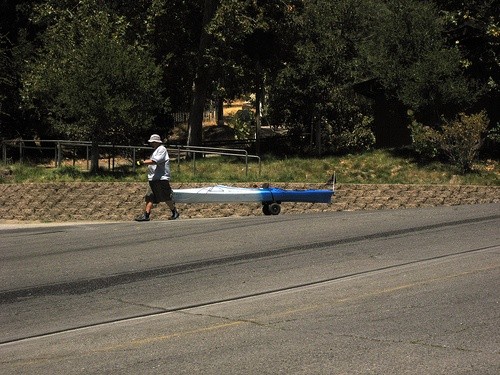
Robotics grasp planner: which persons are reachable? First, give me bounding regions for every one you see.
[134,134,179,221]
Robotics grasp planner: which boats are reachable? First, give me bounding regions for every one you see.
[171,184,334,203]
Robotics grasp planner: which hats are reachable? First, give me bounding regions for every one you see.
[148,134,162,143]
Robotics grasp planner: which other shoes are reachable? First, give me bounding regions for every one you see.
[135,211,150,221]
[168,207,180,220]
